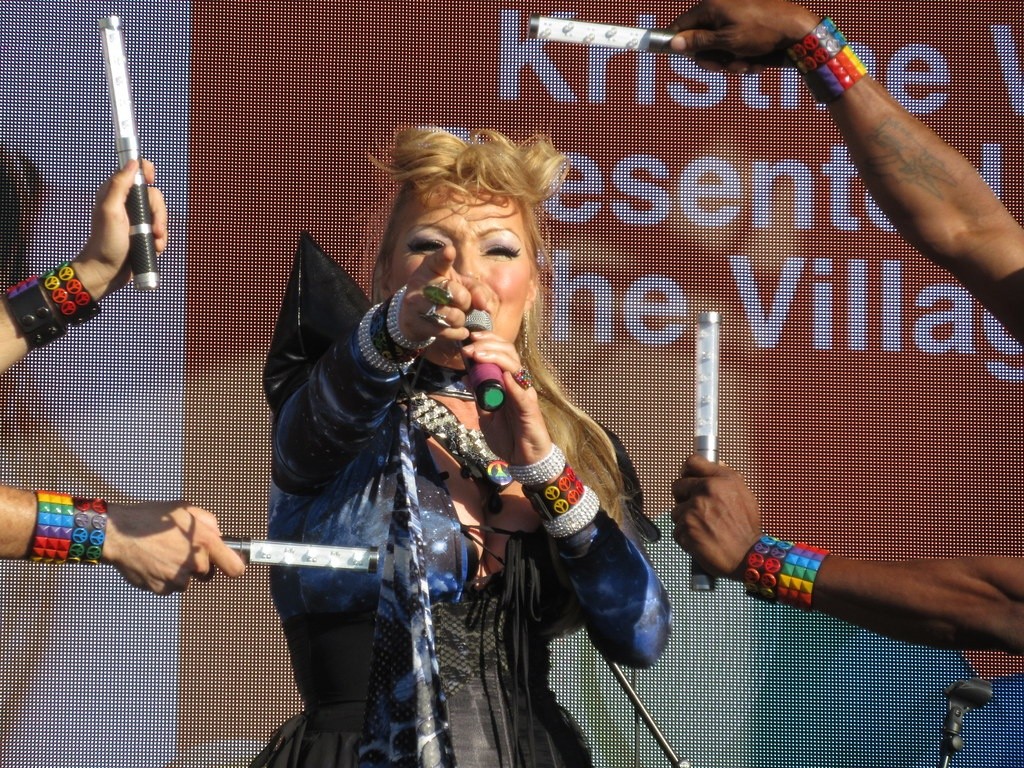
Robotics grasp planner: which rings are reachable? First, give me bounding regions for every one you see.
[513,367,534,391]
[423,279,453,305]
[420,305,451,328]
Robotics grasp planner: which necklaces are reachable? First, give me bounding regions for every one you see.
[413,357,475,401]
[405,388,514,515]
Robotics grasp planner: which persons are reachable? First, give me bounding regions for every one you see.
[667,0,1023,654]
[251,126,671,768]
[0,158,245,595]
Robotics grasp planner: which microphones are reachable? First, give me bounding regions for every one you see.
[454,306,506,413]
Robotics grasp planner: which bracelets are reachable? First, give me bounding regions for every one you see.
[508,442,600,538]
[777,543,830,613]
[787,16,847,75]
[66,496,108,564]
[28,489,75,565]
[801,43,869,103]
[5,276,68,349]
[39,261,102,328]
[745,536,795,605]
[358,284,436,372]
